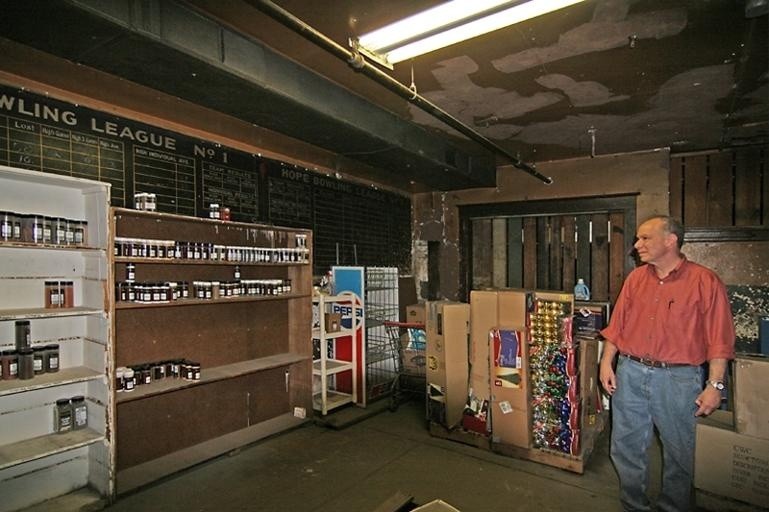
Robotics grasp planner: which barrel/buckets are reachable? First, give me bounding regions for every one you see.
[574,278,590,301]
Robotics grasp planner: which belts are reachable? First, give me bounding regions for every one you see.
[615,349,696,372]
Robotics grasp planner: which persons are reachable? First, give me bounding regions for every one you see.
[597,216,737,511]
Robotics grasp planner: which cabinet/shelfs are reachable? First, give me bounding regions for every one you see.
[0,165,112,511]
[332,267,400,408]
[311,293,358,416]
[109,207,313,505]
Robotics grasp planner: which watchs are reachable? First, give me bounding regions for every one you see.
[706,378,727,391]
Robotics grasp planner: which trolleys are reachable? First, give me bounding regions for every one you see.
[381,320,431,410]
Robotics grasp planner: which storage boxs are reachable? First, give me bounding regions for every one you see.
[399,288,595,450]
[694,410,769,508]
[728,356,769,439]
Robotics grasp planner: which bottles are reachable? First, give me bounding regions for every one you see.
[114,235,311,267]
[1,211,89,246]
[44,280,75,309]
[116,355,201,393]
[209,204,231,222]
[117,263,294,305]
[133,192,159,214]
[0,319,59,381]
[53,395,90,434]
